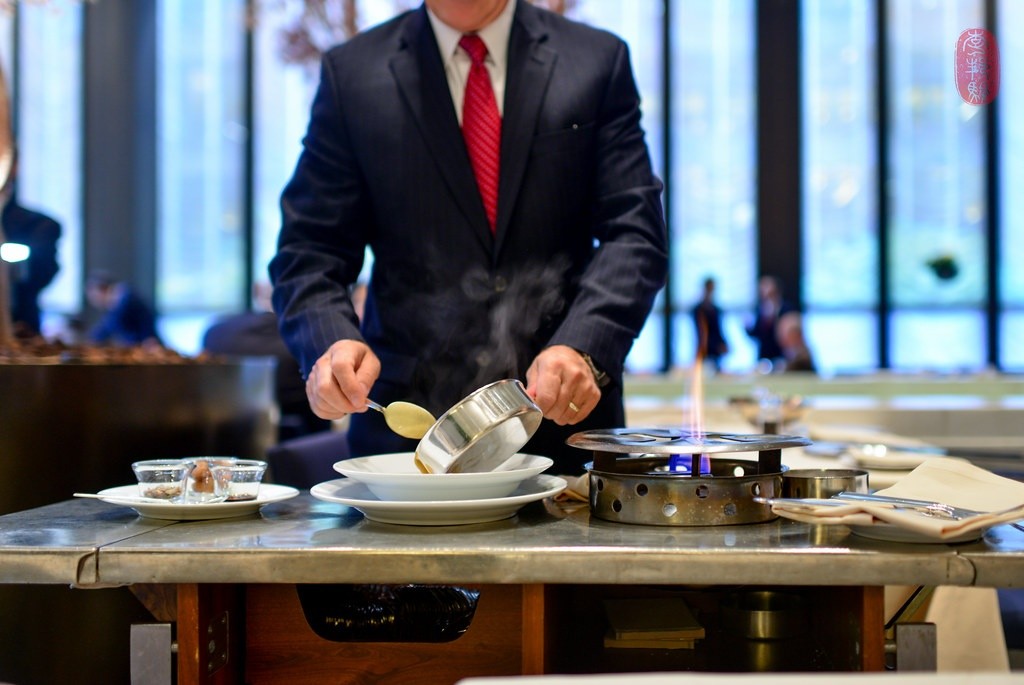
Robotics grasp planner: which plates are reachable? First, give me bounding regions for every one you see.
[843,523,993,543]
[97,484,300,520]
[333,452,554,500]
[310,474,568,525]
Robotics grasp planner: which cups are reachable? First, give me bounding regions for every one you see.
[132,460,194,505]
[225,459,268,501]
[182,456,237,505]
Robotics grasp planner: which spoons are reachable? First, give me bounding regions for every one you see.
[364,397,437,439]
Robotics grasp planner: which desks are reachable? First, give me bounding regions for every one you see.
[0,486,1024,680]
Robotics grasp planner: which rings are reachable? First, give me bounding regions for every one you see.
[569,402,580,413]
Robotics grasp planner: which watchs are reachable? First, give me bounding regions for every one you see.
[581,351,610,388]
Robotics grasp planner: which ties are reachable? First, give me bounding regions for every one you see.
[460,36,502,237]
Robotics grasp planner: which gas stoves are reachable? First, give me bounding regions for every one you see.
[565,425,815,526]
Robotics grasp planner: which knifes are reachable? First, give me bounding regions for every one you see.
[838,491,989,520]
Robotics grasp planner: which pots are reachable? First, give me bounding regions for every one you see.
[415,378,544,474]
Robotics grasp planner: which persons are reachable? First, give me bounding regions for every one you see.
[745,277,792,360]
[775,311,816,373]
[270,0,674,475]
[693,279,728,373]
[84,269,164,346]
[0,148,60,339]
